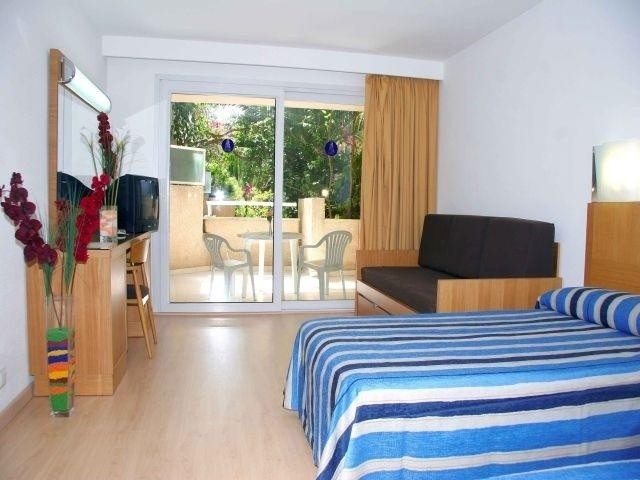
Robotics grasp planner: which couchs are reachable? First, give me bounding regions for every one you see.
[354,214,563,316]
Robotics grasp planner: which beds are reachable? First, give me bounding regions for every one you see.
[297,201,639,479]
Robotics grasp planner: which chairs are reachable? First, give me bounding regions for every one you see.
[297,230,352,300]
[126,237,157,358]
[202,233,257,302]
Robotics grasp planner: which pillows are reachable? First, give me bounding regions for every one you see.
[535,286,640,339]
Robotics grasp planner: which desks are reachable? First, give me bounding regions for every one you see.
[25,231,151,398]
[239,231,304,301]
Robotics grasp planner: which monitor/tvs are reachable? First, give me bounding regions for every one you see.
[57,172,94,207]
[103,174,159,233]
[170,144,206,185]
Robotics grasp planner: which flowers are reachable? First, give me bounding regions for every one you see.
[1,170,110,331]
[79,110,134,210]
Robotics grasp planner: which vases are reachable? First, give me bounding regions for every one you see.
[98,205,118,249]
[44,295,75,418]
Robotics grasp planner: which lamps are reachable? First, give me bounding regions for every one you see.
[61,57,112,116]
